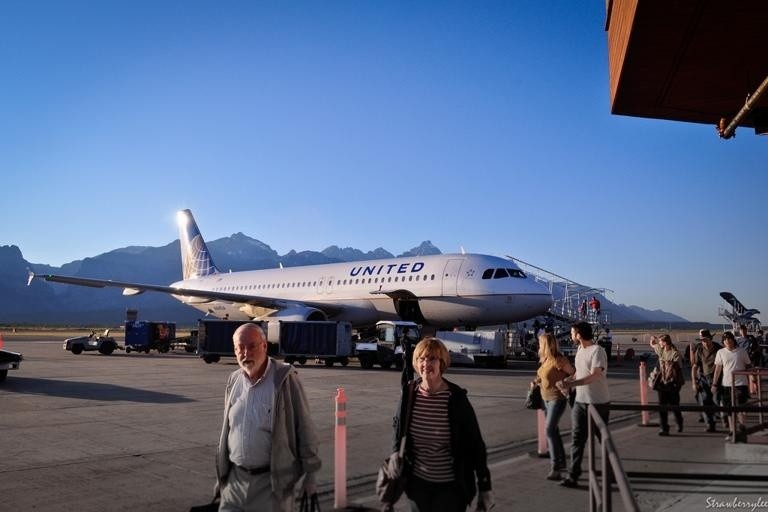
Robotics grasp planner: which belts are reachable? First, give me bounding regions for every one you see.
[228,460,271,475]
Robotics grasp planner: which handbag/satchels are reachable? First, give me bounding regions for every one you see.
[375,379,416,507]
[526,385,542,409]
[647,366,662,390]
[190,490,220,512]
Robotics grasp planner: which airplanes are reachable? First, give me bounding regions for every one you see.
[22,206,562,368]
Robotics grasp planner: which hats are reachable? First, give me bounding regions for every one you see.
[696,329,716,340]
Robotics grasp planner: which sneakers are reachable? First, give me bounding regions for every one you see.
[659,418,733,441]
[560,480,578,488]
[547,470,562,480]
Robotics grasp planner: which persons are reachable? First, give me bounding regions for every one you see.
[521,312,554,342]
[596,329,609,343]
[525,333,577,480]
[649,333,686,436]
[688,325,768,443]
[578,299,587,317]
[555,322,612,487]
[399,326,418,391]
[212,322,321,511]
[602,327,612,359]
[589,297,600,321]
[383,337,494,511]
